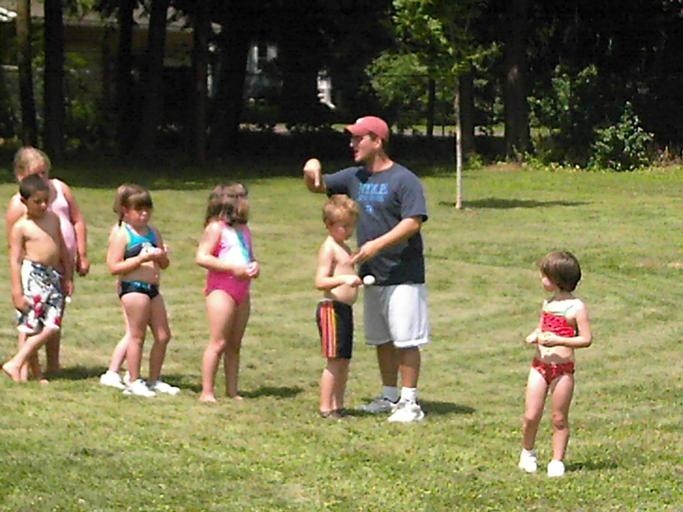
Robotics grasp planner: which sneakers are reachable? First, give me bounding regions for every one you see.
[353,391,402,412]
[121,378,158,398]
[384,398,425,424]
[515,452,539,473]
[146,379,182,397]
[316,405,353,420]
[98,369,126,390]
[545,458,567,478]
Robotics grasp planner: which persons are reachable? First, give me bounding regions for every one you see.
[5,145,89,384]
[315,194,363,422]
[196,179,258,404]
[1,172,74,383]
[517,249,592,477]
[99,181,131,390]
[303,115,429,424]
[107,186,182,398]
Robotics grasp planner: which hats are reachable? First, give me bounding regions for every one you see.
[342,115,391,145]
